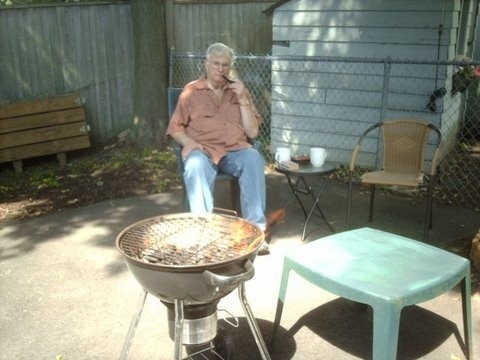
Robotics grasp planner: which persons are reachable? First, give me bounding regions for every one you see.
[165,42,271,256]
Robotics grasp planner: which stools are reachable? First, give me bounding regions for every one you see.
[268,227,473,360]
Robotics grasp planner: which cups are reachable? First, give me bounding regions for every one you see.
[311,148,327,168]
[275,148,290,163]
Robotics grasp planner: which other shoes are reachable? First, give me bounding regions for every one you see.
[257,240,270,255]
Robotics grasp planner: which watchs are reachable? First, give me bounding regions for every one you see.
[239,98,251,107]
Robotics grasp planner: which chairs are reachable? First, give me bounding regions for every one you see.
[344,119,441,243]
[168,88,262,215]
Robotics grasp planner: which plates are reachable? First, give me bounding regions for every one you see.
[291,156,310,163]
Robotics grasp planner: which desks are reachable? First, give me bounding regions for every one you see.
[271,159,336,241]
